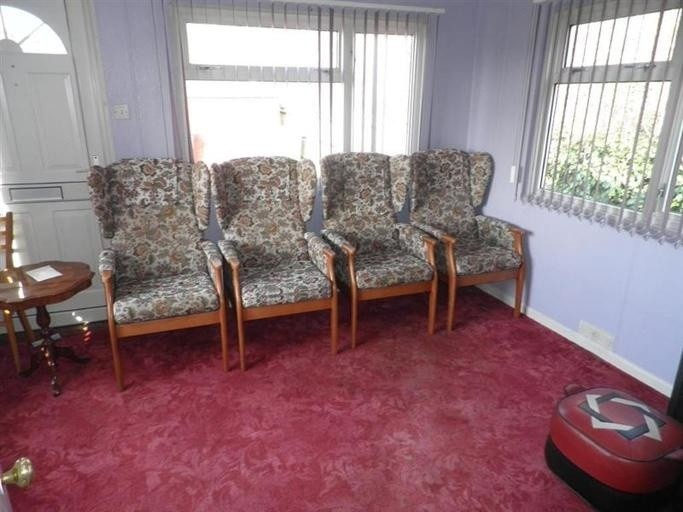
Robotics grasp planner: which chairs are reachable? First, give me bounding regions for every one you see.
[210,155,339,371]
[407,149,525,333]
[319,152,438,350]
[88,156,229,393]
[0,212,35,374]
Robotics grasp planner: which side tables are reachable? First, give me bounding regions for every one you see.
[0,260,96,397]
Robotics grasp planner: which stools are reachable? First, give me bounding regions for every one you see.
[544,383,683,512]
